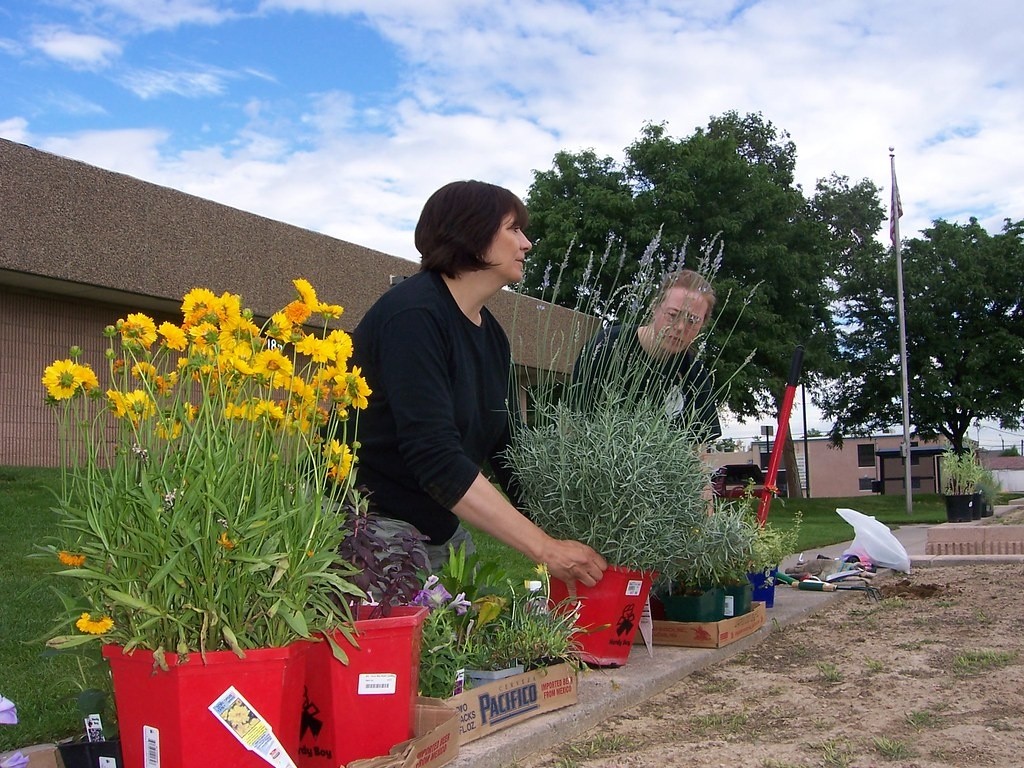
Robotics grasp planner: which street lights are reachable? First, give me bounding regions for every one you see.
[998,434,1004,450]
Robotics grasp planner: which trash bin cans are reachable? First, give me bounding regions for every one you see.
[871,480,882,492]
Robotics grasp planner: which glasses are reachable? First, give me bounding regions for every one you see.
[660,304,704,329]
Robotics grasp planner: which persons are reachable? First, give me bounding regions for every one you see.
[568,269,722,516]
[297,180,607,609]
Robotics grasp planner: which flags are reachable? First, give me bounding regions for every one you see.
[890,163,903,245]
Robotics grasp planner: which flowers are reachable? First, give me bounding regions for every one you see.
[397,538,612,699]
[14,277,370,678]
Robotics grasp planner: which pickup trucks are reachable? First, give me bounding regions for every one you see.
[709,464,781,513]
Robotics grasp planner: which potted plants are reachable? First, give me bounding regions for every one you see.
[649,499,756,623]
[492,378,716,666]
[299,488,429,768]
[737,477,802,609]
[979,461,1004,518]
[464,619,524,688]
[38,585,123,768]
[972,459,984,520]
[939,435,978,523]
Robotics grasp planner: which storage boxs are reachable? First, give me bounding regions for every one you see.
[415,660,580,747]
[633,601,767,649]
[337,703,461,768]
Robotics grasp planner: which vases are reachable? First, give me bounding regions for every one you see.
[101,641,310,768]
